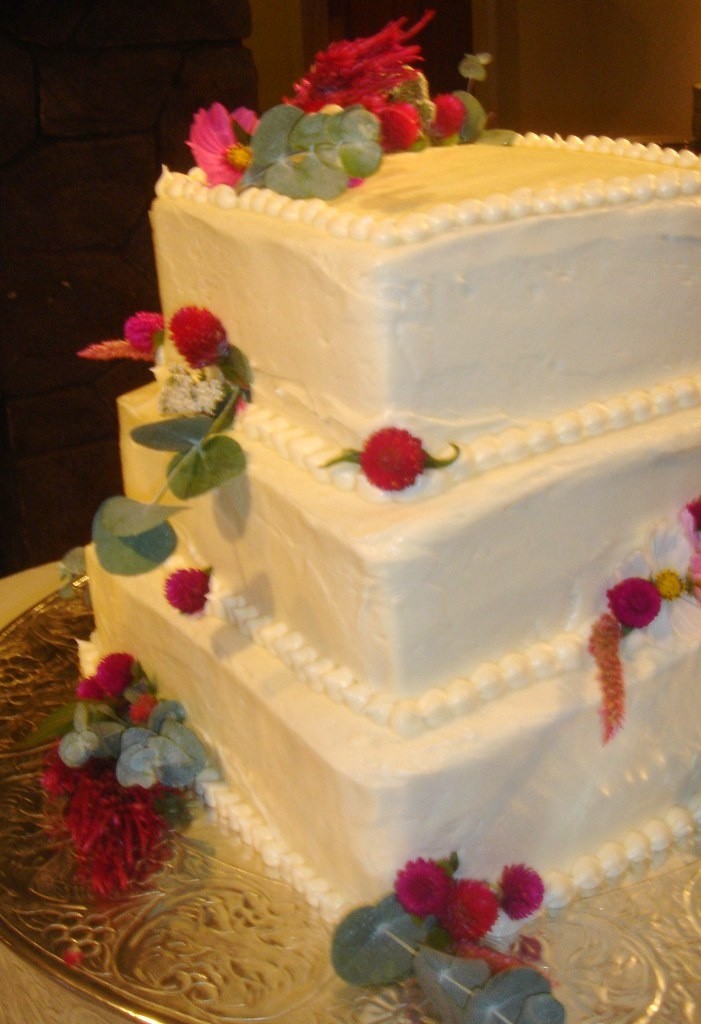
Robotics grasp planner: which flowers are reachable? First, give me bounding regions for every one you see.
[162,566,212,615]
[184,7,493,202]
[588,496,701,746]
[332,852,566,1024]
[77,310,254,578]
[13,652,209,899]
[319,427,461,490]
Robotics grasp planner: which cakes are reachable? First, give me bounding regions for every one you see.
[38,12,700,989]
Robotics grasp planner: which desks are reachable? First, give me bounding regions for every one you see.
[0,561,701,1024]
